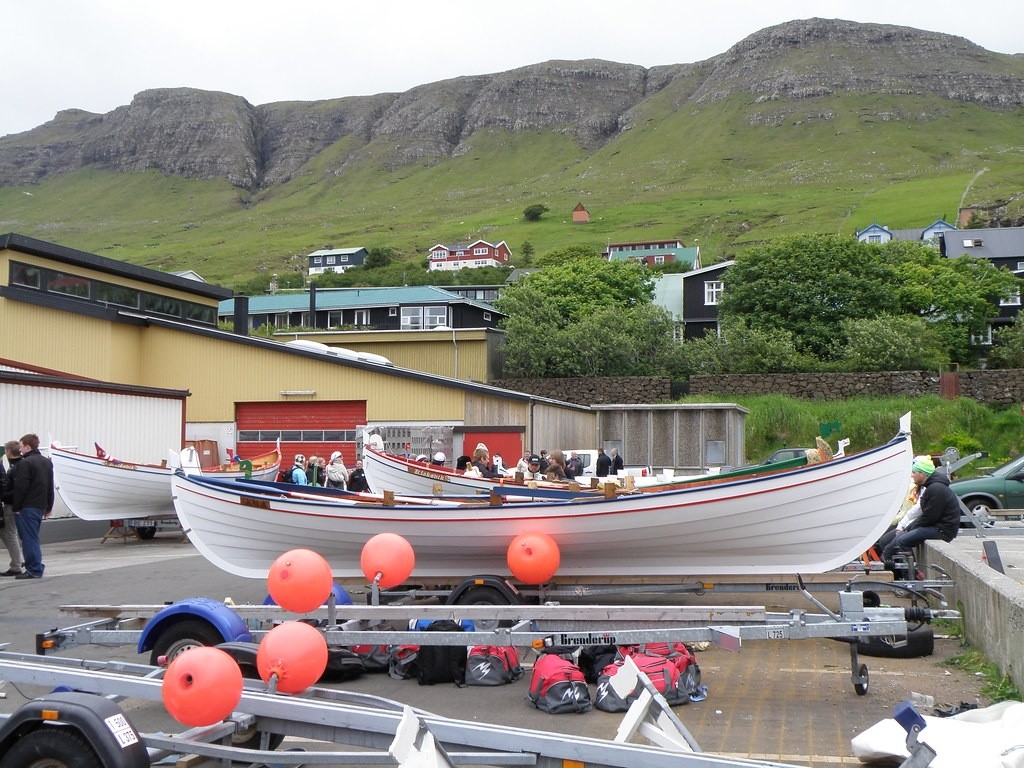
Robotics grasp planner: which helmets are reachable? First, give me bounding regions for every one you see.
[476,443,488,451]
[416,454,428,462]
[309,456,317,462]
[331,450,342,460]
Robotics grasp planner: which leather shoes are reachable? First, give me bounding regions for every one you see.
[21,561,26,567]
[15,571,33,578]
[0,569,23,576]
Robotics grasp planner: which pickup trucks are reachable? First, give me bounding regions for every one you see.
[506,449,653,485]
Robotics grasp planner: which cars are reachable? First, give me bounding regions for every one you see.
[948,456,1024,529]
[730,449,810,472]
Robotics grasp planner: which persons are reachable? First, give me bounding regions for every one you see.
[292,442,624,493]
[0,434,54,580]
[870,455,961,580]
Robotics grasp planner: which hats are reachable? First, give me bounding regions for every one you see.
[366,425,376,434]
[912,455,935,476]
[434,452,446,462]
[528,454,540,465]
[295,454,305,463]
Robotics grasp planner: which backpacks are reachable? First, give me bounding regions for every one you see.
[282,466,298,484]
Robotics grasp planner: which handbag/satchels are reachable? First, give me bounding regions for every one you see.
[351,618,702,715]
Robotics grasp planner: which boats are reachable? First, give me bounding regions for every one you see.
[360,431,850,497]
[170,411,913,578]
[49,437,282,521]
